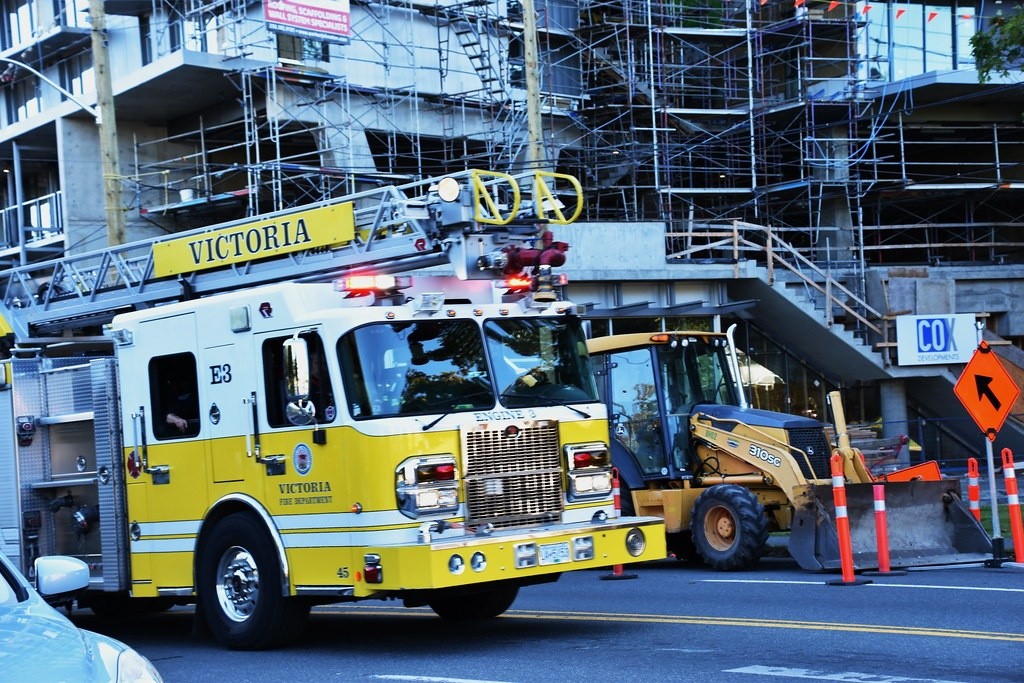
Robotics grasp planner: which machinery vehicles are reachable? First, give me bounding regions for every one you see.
[0,169,680,652]
[587,332,1011,572]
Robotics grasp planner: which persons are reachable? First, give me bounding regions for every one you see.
[150,369,188,433]
[397,331,464,399]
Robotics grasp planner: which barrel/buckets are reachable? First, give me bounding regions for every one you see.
[180,189,193,200]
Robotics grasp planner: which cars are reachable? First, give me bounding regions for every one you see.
[0,553,164,682]
